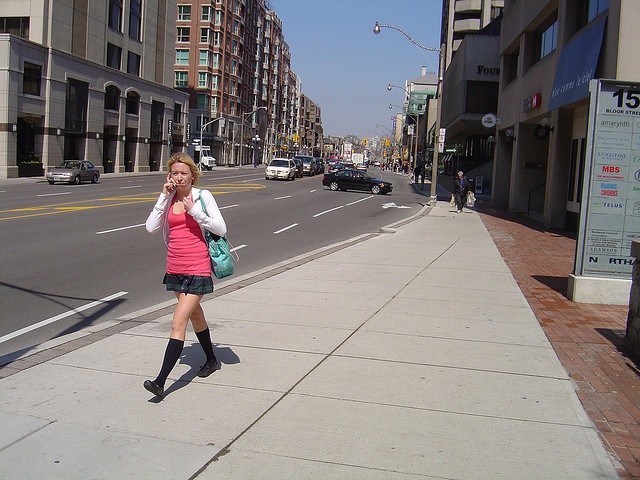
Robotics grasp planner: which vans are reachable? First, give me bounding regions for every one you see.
[195,146,217,171]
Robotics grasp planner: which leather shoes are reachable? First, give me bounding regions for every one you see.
[143,379,165,398]
[196,360,218,377]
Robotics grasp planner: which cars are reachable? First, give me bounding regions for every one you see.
[292,159,303,178]
[356,163,367,172]
[328,164,345,174]
[322,170,394,195]
[46,160,100,185]
[265,158,297,181]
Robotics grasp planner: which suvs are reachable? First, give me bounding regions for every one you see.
[294,155,317,176]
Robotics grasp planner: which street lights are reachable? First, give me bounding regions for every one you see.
[390,115,415,174]
[240,107,268,166]
[373,21,446,207]
[388,104,419,174]
[387,84,430,184]
[197,114,224,172]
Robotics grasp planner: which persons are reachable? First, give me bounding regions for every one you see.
[414,165,420,184]
[143,151,228,397]
[453,171,472,214]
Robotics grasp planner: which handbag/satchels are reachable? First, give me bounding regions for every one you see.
[198,188,240,280]
